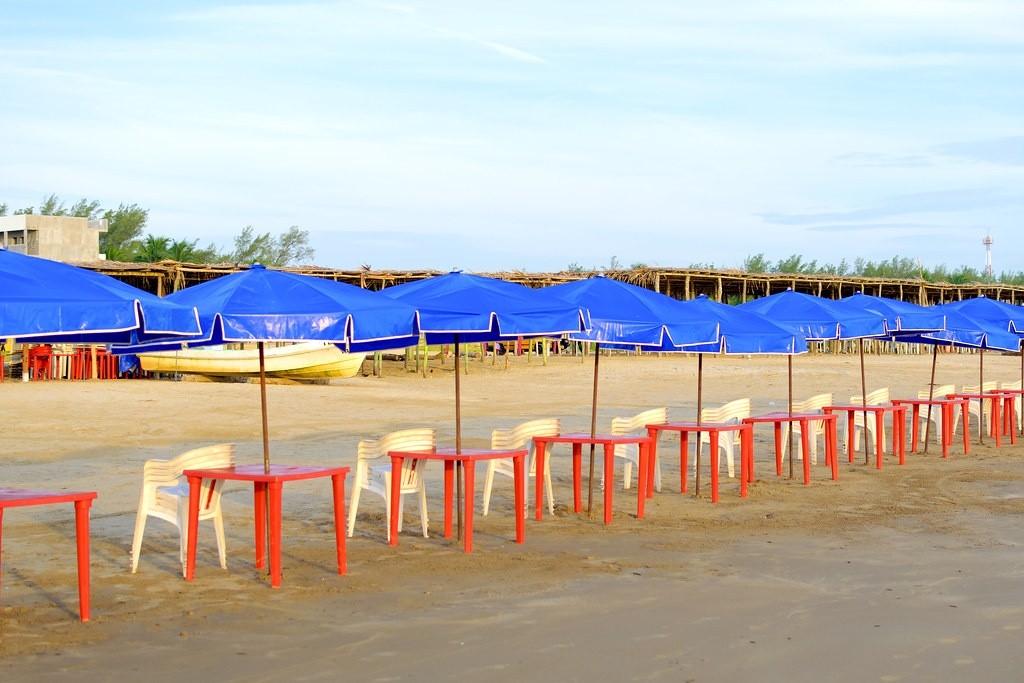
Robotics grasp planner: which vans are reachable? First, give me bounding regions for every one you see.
[487,336,552,356]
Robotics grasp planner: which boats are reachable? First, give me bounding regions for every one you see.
[135,341,366,381]
[365,341,483,361]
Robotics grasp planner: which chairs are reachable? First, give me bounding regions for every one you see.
[134,443,237,579]
[600,407,669,493]
[483,418,560,519]
[348,428,437,541]
[782,393,834,465]
[696,398,752,477]
[910,381,1022,444]
[843,388,890,454]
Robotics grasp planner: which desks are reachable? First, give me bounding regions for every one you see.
[183,464,351,587]
[822,405,907,468]
[990,389,1024,438]
[946,394,1016,446]
[891,399,970,465]
[0,489,98,621]
[28,346,151,379]
[743,414,837,484]
[644,422,748,503]
[533,433,652,525]
[387,448,528,552]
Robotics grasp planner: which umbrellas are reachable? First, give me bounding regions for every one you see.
[832,291,947,465]
[530,271,719,511]
[334,270,591,541]
[108,263,420,574]
[0,248,202,345]
[944,295,1024,444]
[597,294,807,496]
[875,303,1022,455]
[732,286,888,479]
